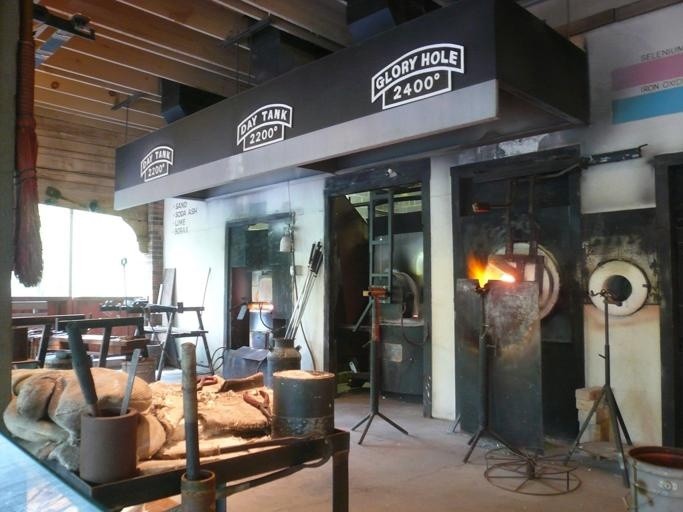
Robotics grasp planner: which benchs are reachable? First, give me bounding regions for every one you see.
[28,333,151,362]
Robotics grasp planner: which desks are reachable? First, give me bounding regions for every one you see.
[0,417,351,512]
[99,303,215,381]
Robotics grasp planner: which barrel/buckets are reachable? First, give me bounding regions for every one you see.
[625,449,682,512]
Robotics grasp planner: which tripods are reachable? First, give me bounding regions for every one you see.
[564,296,632,488]
[463,333,526,464]
[351,299,409,444]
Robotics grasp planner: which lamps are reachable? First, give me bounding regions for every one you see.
[279,225,293,253]
